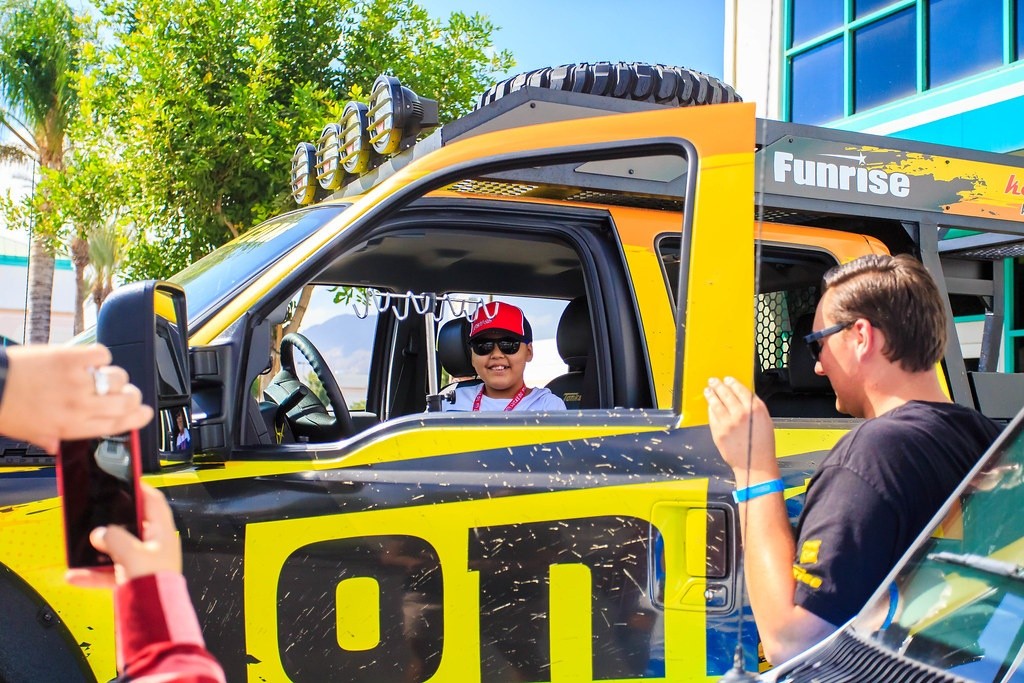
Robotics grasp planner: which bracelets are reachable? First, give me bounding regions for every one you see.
[732,477,784,504]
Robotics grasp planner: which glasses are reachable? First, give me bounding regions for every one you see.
[469,336,530,355]
[802,318,882,362]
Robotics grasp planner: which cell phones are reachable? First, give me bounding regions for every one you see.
[56,429,146,569]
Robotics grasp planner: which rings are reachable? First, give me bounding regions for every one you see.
[95,370,110,395]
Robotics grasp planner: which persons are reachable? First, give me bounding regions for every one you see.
[1,342,232,682]
[702,253,1008,666]
[424,302,567,412]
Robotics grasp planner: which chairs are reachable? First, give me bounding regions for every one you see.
[764,312,855,419]
[543,296,601,410]
[438,315,484,391]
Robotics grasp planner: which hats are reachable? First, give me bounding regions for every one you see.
[469,302,533,341]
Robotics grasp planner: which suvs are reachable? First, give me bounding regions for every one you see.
[0,61,1024,683]
[722,409,1024,683]
